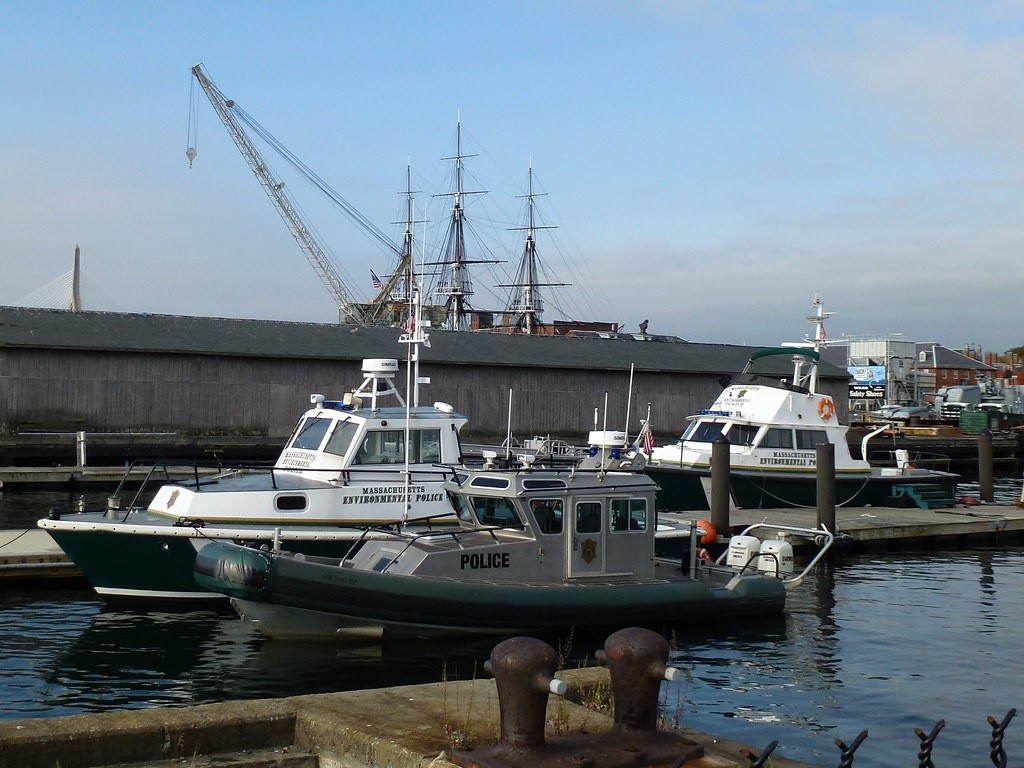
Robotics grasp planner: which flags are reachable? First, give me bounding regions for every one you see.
[371,271,383,288]
[401,306,416,337]
[643,429,655,454]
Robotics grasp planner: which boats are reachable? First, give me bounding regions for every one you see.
[35,359,645,613]
[629,290,961,513]
[186,63,687,344]
[191,385,837,638]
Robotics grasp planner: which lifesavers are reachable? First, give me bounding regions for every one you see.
[816,397,835,419]
